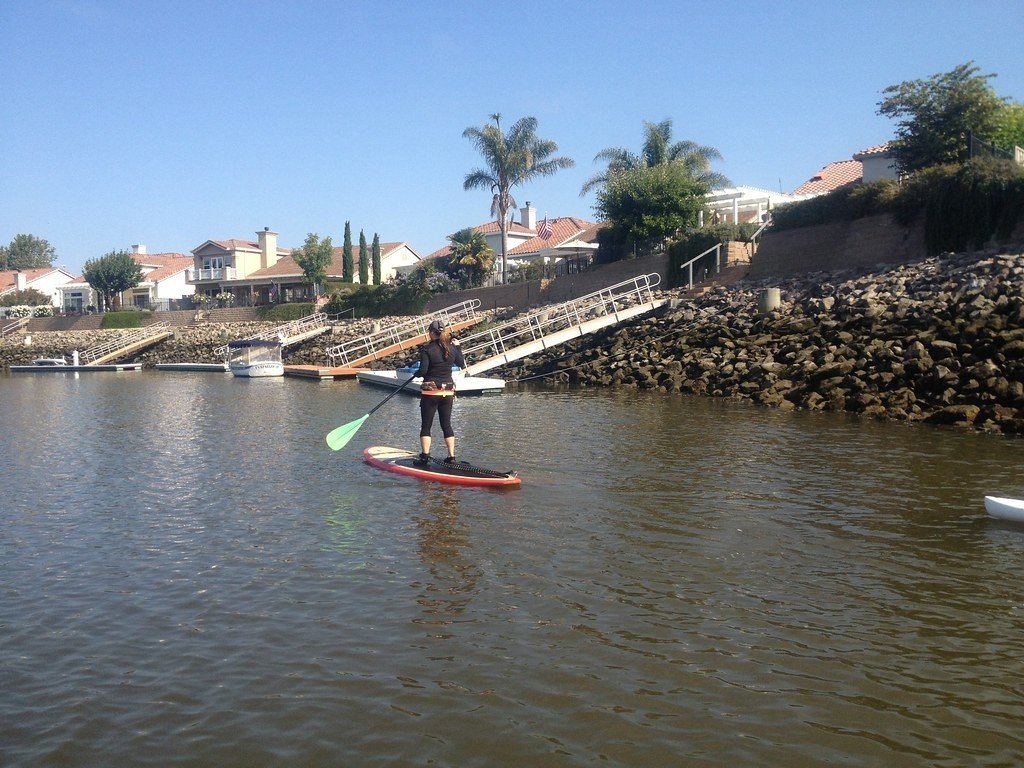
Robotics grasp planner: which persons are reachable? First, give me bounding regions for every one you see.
[413,321,465,469]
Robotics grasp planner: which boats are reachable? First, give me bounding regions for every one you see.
[984,495,1024,524]
[31,354,68,366]
[228,338,286,379]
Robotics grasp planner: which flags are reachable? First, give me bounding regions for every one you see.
[537,217,553,241]
[270,282,277,296]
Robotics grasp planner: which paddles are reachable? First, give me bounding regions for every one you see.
[325,337,456,451]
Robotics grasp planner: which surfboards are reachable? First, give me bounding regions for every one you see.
[363,445,523,487]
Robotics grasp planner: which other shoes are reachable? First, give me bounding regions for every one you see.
[444,457,456,464]
[413,458,428,467]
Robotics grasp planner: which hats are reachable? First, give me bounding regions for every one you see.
[428,321,444,332]
